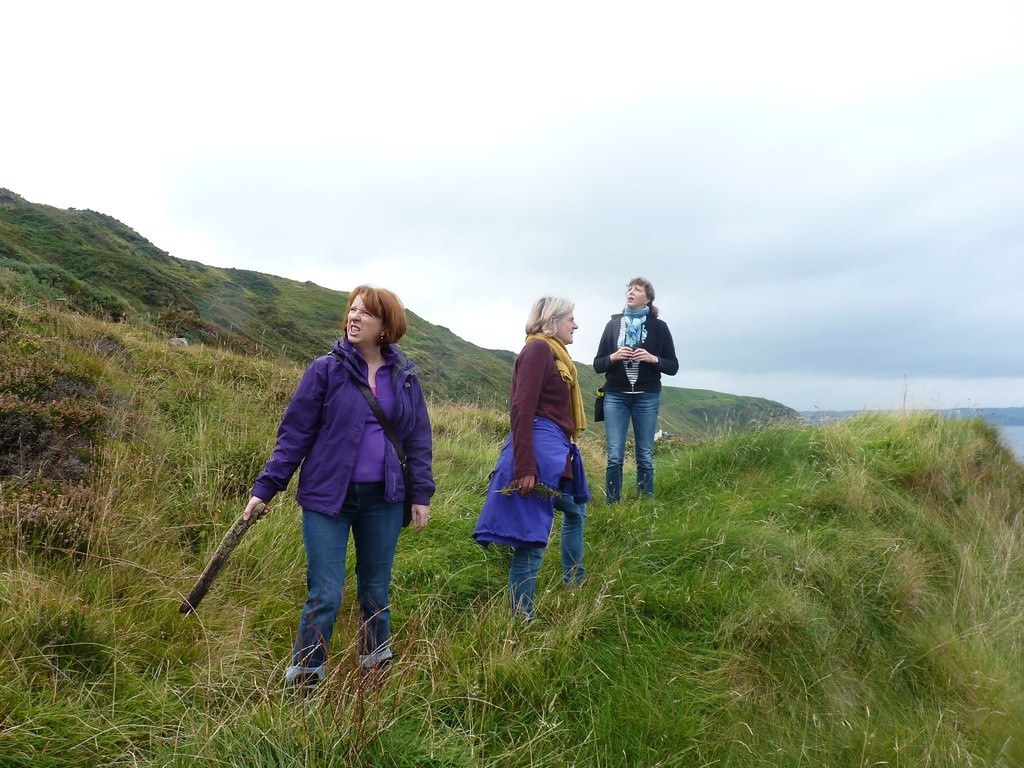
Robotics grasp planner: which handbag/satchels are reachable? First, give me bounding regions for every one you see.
[595,388,606,422]
[401,461,412,528]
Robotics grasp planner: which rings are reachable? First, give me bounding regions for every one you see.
[428,515,431,520]
[518,485,522,489]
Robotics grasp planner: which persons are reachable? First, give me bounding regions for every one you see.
[594,278,678,505]
[243,286,435,697]
[471,298,592,617]
